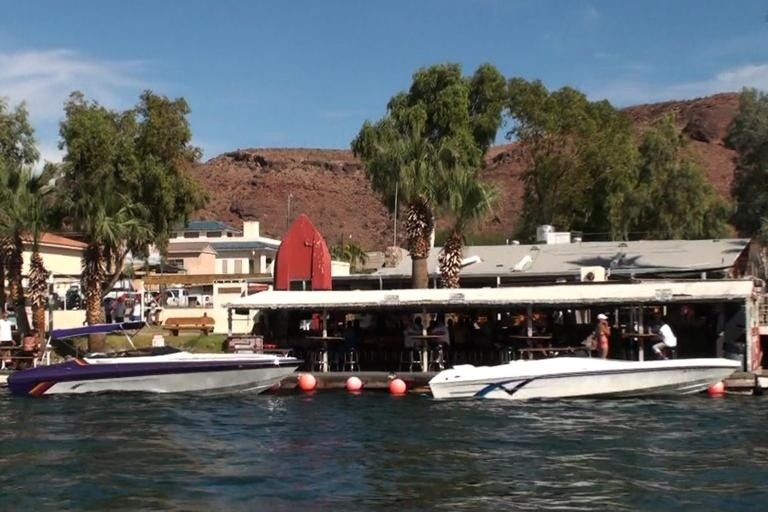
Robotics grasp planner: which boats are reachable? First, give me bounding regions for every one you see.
[7,321,306,397]
[428,357,742,402]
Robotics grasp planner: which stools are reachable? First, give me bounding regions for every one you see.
[338,346,361,371]
[399,345,423,374]
[304,347,318,369]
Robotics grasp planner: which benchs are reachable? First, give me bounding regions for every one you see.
[163,317,215,337]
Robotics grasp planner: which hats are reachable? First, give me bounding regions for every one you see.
[596,312,609,320]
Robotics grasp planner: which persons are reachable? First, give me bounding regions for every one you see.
[0,313,18,346]
[594,314,613,359]
[344,309,507,373]
[104,294,142,324]
[651,316,678,360]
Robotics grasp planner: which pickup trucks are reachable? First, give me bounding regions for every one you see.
[64,279,149,309]
[155,288,211,306]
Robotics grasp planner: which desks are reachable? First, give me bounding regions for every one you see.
[413,330,441,344]
[1,341,43,369]
[509,332,554,345]
[617,330,659,344]
[303,329,348,348]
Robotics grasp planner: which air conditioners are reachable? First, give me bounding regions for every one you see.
[581,266,608,282]
